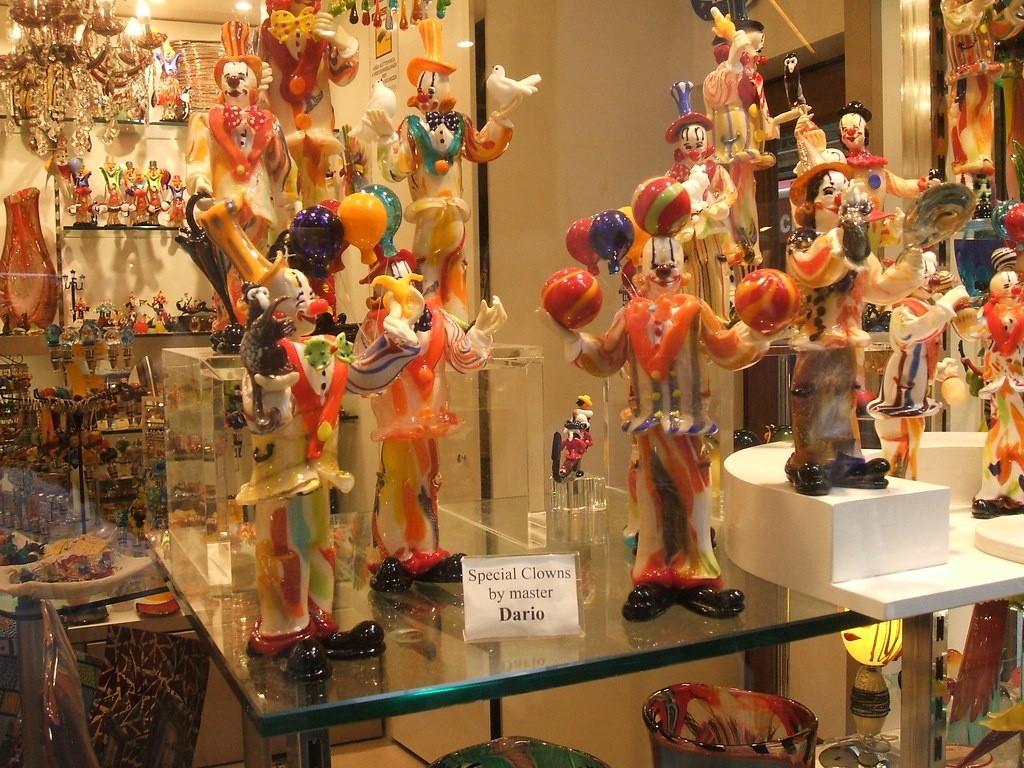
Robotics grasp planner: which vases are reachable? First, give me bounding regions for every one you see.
[0,187,57,332]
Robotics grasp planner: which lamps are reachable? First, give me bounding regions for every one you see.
[0,0,167,168]
[841,618,903,745]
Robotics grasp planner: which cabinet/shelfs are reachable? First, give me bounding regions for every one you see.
[49,116,216,341]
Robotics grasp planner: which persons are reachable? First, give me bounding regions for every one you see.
[351,248,509,595]
[55,51,194,334]
[183,1,517,347]
[533,236,798,624]
[236,266,425,680]
[550,1,1024,521]
